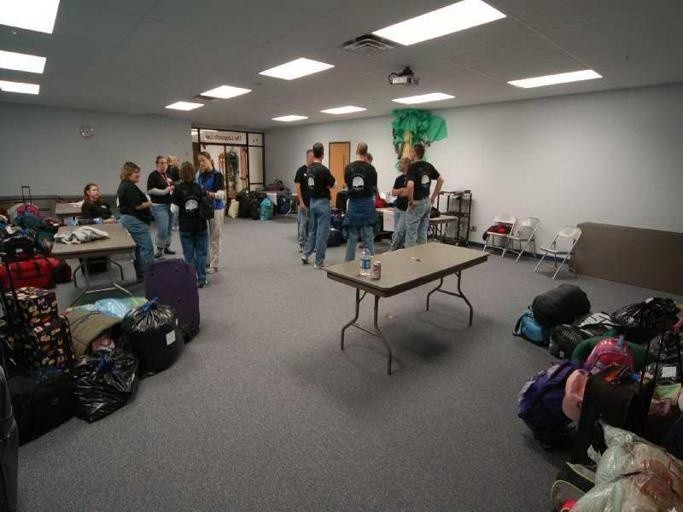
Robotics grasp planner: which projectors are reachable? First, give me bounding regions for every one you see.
[390,72,421,86]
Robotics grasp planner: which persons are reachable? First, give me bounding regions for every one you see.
[147,156,176,258]
[294,149,317,253]
[81,184,111,220]
[363,154,373,164]
[301,142,336,269]
[169,161,210,289]
[166,154,181,231]
[194,151,225,274]
[405,144,444,249]
[117,162,154,280]
[344,141,377,262]
[391,158,412,252]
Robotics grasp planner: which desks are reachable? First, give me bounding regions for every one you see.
[279,192,300,218]
[318,238,491,377]
[52,200,86,221]
[49,220,142,312]
[372,206,459,246]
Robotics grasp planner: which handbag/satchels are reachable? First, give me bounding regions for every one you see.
[0,244,73,295]
[227,198,240,219]
[258,198,275,221]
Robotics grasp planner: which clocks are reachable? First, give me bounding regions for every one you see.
[78,125,95,139]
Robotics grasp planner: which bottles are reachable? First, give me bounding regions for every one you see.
[360,244,373,277]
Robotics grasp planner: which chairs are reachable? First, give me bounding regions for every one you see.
[482,212,518,259]
[531,224,582,281]
[505,216,541,266]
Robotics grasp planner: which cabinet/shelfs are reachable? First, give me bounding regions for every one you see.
[435,188,473,247]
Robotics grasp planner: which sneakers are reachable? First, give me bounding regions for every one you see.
[299,246,330,270]
[198,265,216,287]
[154,248,176,258]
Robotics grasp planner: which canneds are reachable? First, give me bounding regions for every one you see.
[373,261,381,279]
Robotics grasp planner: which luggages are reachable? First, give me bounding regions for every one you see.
[0,253,74,369]
[144,258,204,340]
[16,185,39,218]
[576,316,668,470]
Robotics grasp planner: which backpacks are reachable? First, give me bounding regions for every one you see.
[515,361,593,447]
[512,282,683,385]
